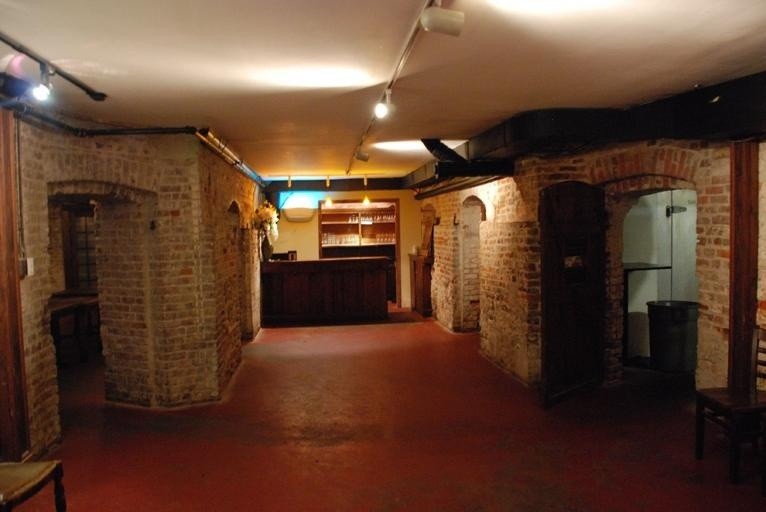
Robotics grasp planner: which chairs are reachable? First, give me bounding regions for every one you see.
[694,322,765,486]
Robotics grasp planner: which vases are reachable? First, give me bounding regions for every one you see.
[261,234,274,260]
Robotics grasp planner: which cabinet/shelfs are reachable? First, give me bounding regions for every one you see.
[318,198,401,305]
[409,255,432,316]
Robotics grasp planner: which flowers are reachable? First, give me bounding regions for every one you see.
[251,201,281,259]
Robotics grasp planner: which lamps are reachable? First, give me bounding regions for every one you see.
[343,0,467,174]
[0,31,108,110]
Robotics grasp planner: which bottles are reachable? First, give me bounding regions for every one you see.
[322,231,394,245]
[347,213,394,222]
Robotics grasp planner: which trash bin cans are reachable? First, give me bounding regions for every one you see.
[647,299,696,395]
